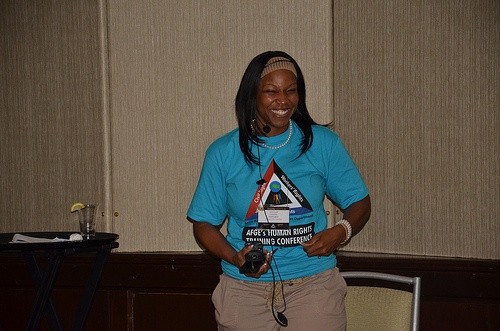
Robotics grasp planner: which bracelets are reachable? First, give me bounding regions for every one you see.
[335,219,352,244]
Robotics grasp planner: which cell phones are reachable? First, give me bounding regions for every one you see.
[239,262,263,274]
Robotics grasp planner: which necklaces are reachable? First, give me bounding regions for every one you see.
[251,118,293,149]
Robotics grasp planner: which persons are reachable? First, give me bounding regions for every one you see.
[187,51,372,331]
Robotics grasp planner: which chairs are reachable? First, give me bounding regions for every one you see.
[339,272,421,330]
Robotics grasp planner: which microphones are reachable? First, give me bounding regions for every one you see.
[256,114,271,133]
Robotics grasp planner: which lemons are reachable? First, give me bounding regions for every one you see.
[70,203,85,212]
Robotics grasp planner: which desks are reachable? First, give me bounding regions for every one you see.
[0,231,119,331]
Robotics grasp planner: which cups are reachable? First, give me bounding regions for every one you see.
[79,205,95,237]
